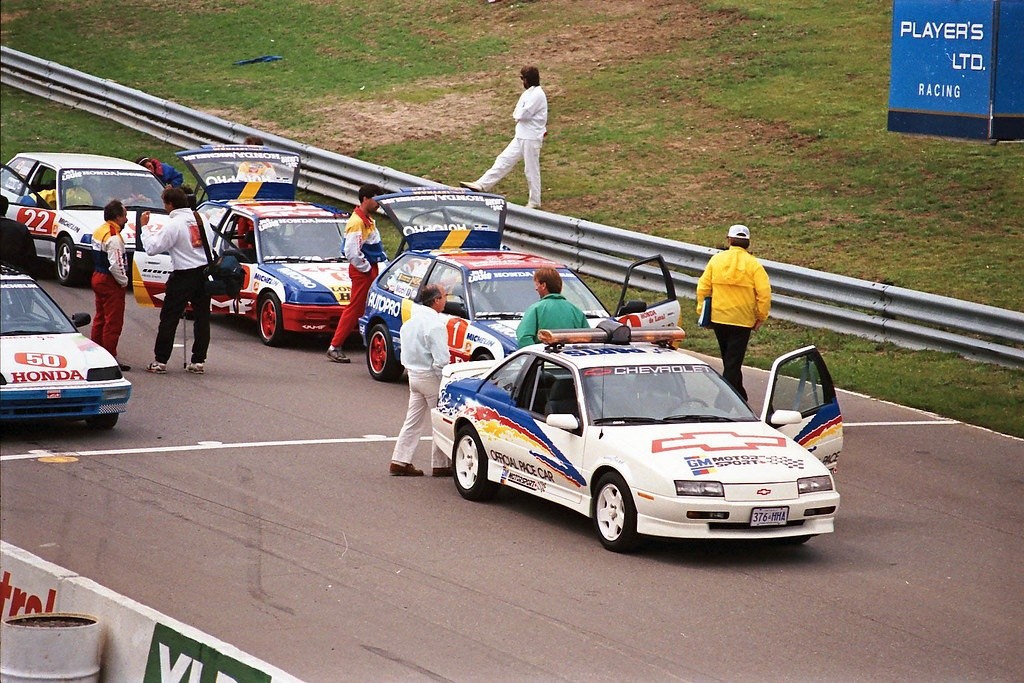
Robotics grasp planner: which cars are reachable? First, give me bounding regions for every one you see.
[0,267,131,429]
[0,152,180,284]
[427,321,843,553]
[175,146,393,348]
[356,187,683,382]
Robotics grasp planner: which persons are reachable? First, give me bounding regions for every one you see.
[0,195,38,313]
[141,188,214,374]
[515,268,591,350]
[460,65,548,208]
[389,283,454,478]
[325,184,388,363]
[135,157,183,189]
[92,200,132,372]
[696,224,772,414]
[236,136,276,248]
[20,186,100,209]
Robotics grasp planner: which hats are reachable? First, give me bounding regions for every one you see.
[728,225,750,239]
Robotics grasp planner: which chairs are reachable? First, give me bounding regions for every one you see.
[528,370,579,417]
[635,372,681,415]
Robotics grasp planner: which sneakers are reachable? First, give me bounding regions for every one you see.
[389,463,424,476]
[186,362,204,374]
[326,348,350,363]
[432,467,453,477]
[145,362,166,374]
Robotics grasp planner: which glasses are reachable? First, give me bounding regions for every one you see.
[520,75,524,80]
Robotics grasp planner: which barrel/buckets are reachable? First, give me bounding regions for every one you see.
[0,613,104,683]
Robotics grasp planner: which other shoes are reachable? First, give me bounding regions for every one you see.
[524,204,541,210]
[120,364,131,371]
[460,181,483,192]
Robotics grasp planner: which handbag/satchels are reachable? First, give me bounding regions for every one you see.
[203,256,246,298]
